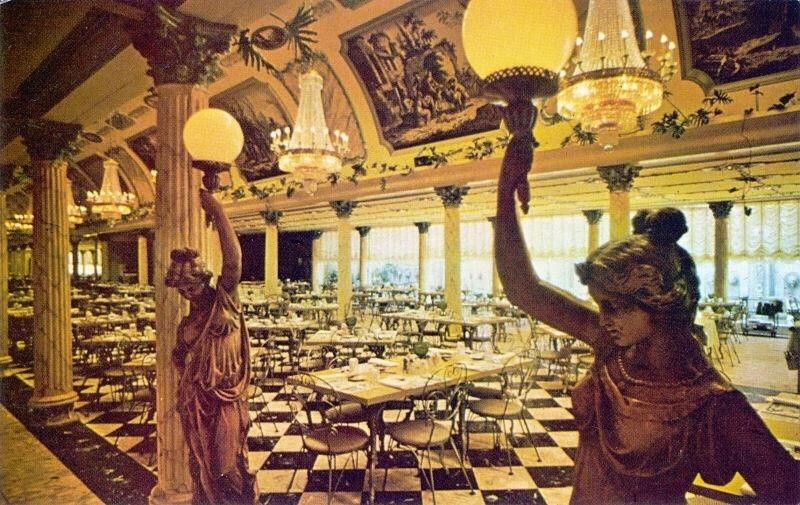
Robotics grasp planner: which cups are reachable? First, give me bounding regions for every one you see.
[280,316,286,325]
[413,342,429,359]
[128,323,136,337]
[348,358,359,373]
[456,340,466,357]
[145,326,154,340]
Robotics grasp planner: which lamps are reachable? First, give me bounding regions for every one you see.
[6,125,140,244]
[269,69,350,198]
[460,0,579,216]
[543,0,679,150]
[182,106,247,227]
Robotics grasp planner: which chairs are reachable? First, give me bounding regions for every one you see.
[70,278,157,471]
[238,280,594,503]
[8,277,36,368]
[695,296,749,383]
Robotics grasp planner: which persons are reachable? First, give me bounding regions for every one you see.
[493,131,800,505]
[162,189,258,504]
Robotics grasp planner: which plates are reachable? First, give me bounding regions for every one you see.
[430,348,457,355]
[457,363,493,372]
[381,376,427,390]
